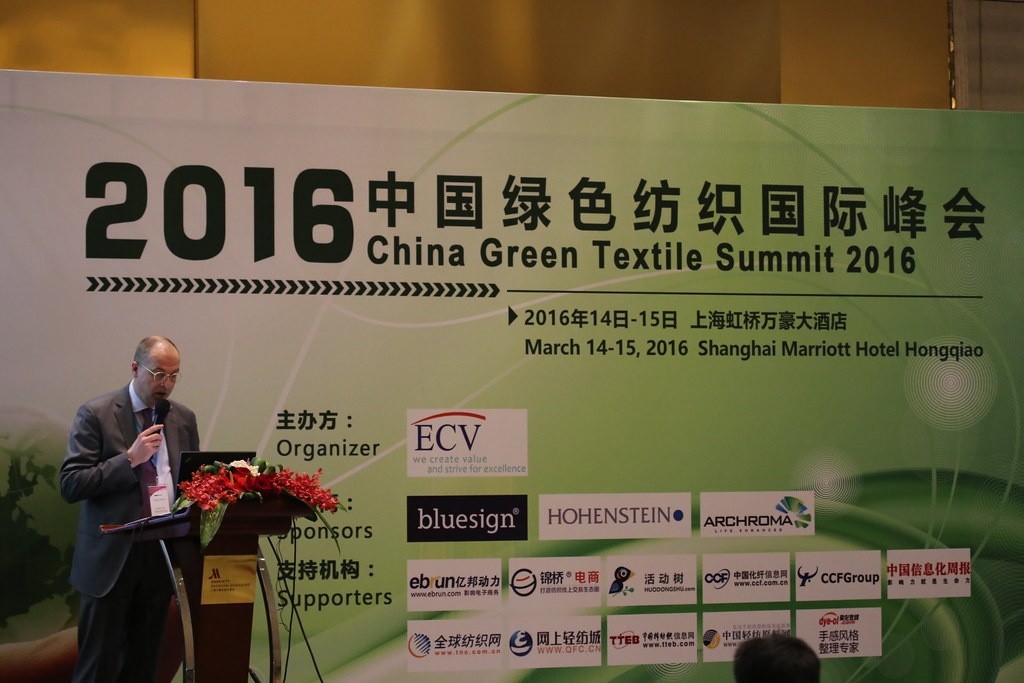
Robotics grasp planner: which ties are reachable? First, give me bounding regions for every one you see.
[139,407,156,517]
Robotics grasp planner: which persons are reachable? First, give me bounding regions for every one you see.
[733,633,821,683]
[59,334,201,683]
[0,596,185,683]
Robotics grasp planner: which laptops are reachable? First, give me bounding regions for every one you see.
[125,451,257,526]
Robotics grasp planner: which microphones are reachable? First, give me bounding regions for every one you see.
[152,399,171,436]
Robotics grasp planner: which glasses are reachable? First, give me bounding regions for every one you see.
[137,362,182,384]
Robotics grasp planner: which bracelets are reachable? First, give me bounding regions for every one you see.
[128,458,132,466]
